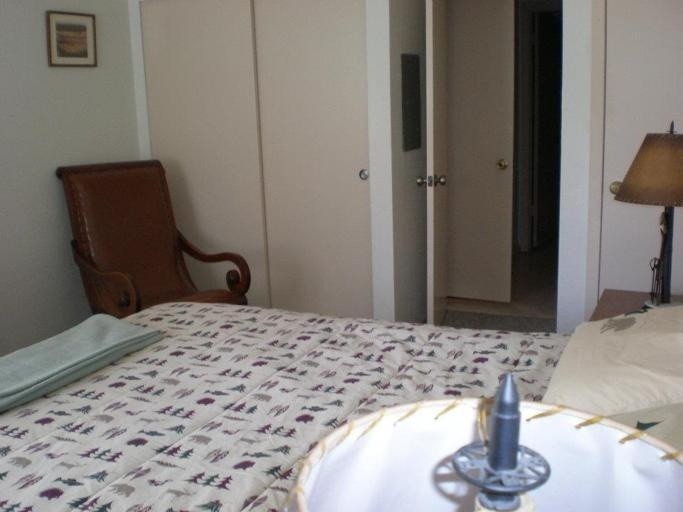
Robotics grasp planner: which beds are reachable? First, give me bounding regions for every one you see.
[0,289,683,512]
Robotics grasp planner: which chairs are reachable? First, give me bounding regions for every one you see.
[53,156,252,319]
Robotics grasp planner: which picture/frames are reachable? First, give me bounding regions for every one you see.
[45,9,99,68]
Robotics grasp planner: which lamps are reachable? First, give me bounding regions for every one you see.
[611,120,682,312]
[287,370,683,510]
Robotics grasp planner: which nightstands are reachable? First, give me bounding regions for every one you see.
[587,285,681,330]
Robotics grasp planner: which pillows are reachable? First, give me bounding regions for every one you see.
[543,311,683,417]
[609,401,683,457]
